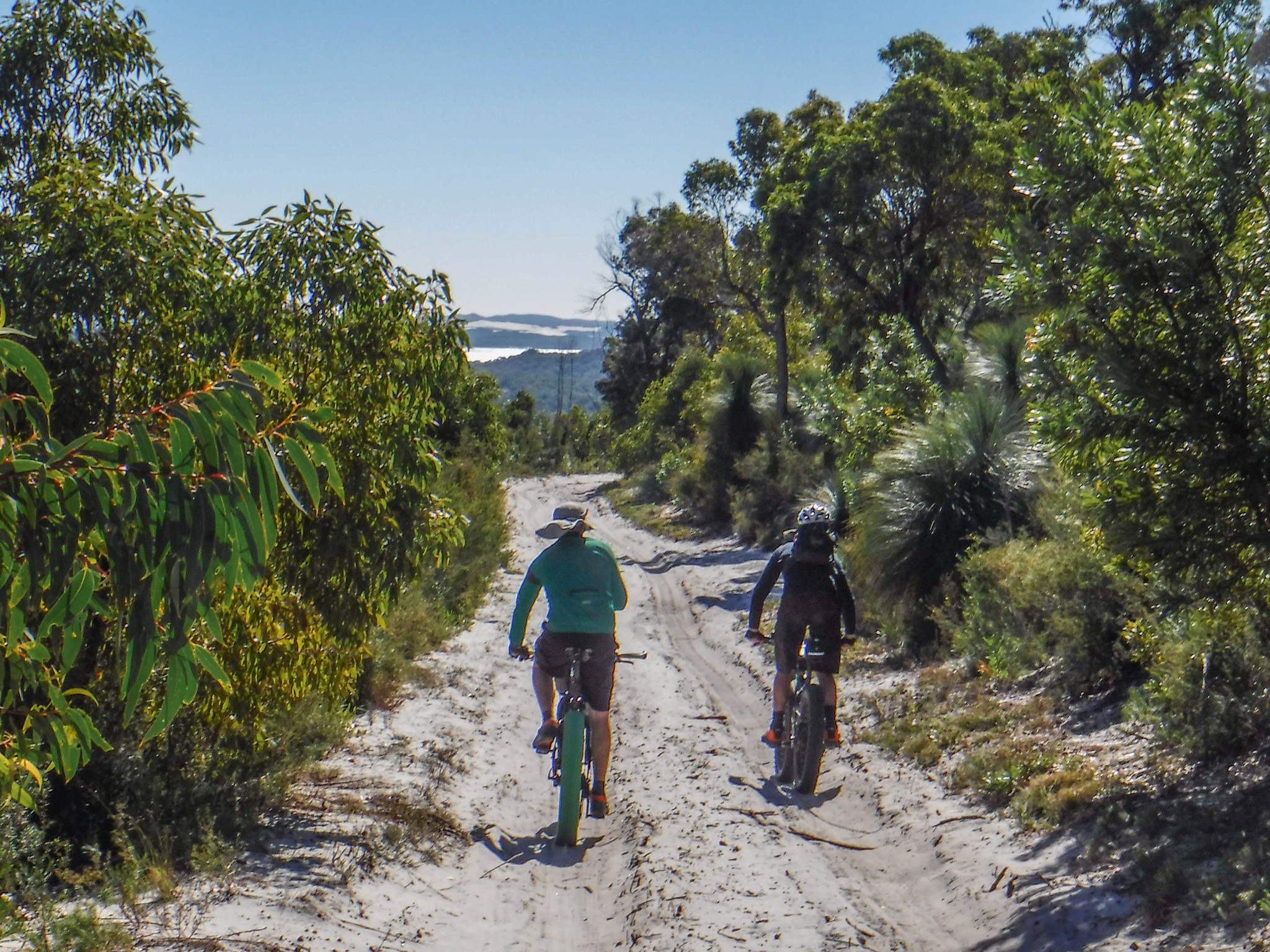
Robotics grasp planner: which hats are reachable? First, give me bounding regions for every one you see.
[535,501,595,539]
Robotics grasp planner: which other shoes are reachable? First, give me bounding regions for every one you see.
[761,729,781,747]
[533,718,558,750]
[824,722,840,747]
[590,792,609,819]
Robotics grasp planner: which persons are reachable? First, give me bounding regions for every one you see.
[745,506,859,751]
[506,504,628,819]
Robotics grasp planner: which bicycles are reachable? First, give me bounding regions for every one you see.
[745,624,856,795]
[510,636,647,847]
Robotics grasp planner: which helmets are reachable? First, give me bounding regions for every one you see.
[797,503,830,524]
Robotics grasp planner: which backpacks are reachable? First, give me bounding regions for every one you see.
[783,538,843,596]
